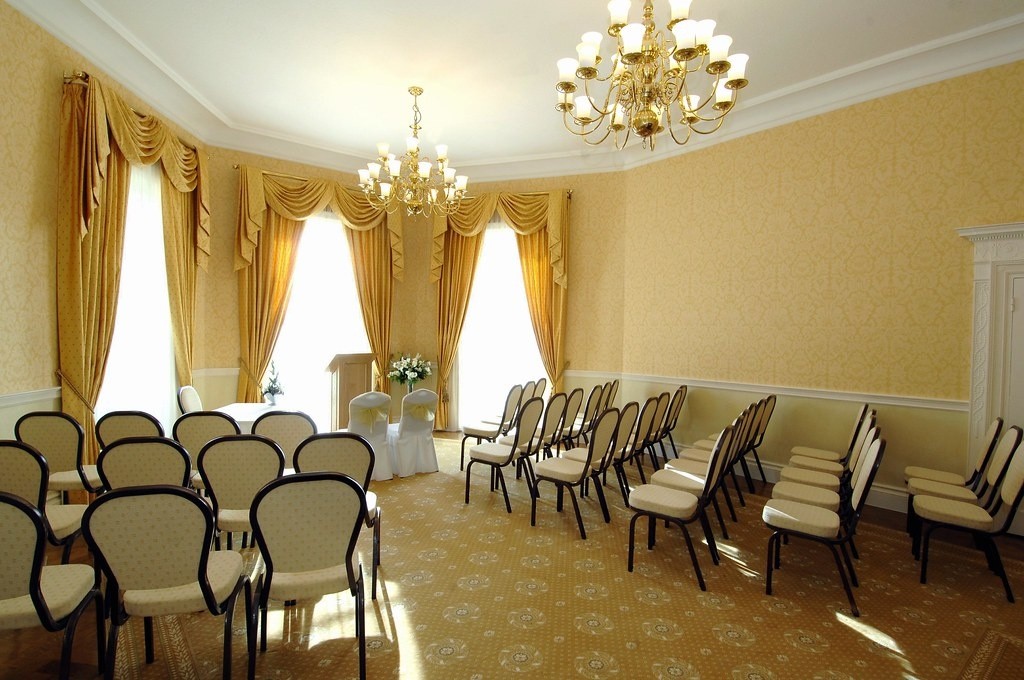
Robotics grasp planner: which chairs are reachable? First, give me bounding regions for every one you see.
[0,378,1024,680]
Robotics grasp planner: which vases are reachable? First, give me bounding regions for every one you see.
[407,381,413,394]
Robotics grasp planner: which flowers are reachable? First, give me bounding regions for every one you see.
[386,352,432,394]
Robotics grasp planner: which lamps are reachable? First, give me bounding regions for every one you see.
[555,0,749,151]
[357,86,468,223]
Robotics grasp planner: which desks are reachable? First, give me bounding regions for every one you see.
[211,403,285,434]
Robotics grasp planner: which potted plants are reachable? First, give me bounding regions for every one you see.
[263,360,284,403]
